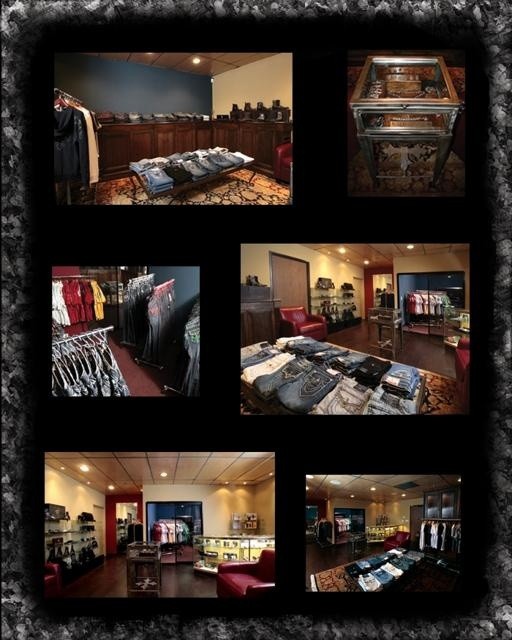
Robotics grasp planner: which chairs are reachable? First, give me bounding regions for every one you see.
[274,306,328,344]
[382,530,410,552]
[215,548,276,598]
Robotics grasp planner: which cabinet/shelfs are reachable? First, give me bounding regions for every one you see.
[193,533,275,576]
[97,121,291,185]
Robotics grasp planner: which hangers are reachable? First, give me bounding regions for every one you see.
[52,326,115,391]
[54,89,80,110]
